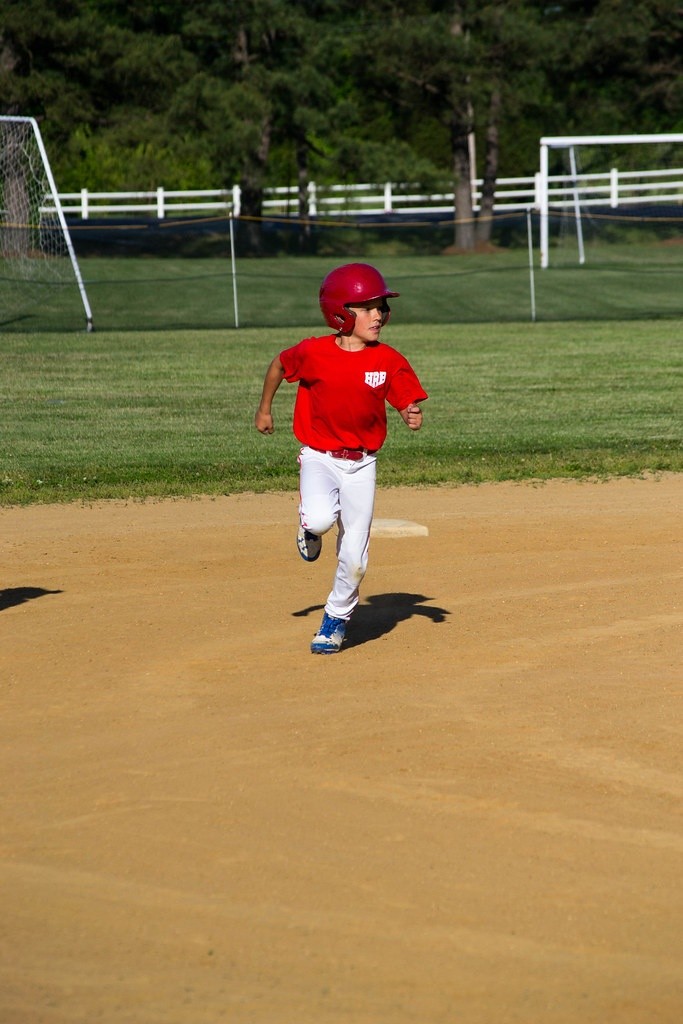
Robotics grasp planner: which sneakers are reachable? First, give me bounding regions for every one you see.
[311,611,348,654]
[297,519,322,561]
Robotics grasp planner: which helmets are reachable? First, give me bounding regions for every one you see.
[318,263,400,337]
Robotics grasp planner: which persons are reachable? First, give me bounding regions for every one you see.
[255,264,429,654]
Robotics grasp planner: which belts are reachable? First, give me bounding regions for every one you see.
[309,445,375,461]
